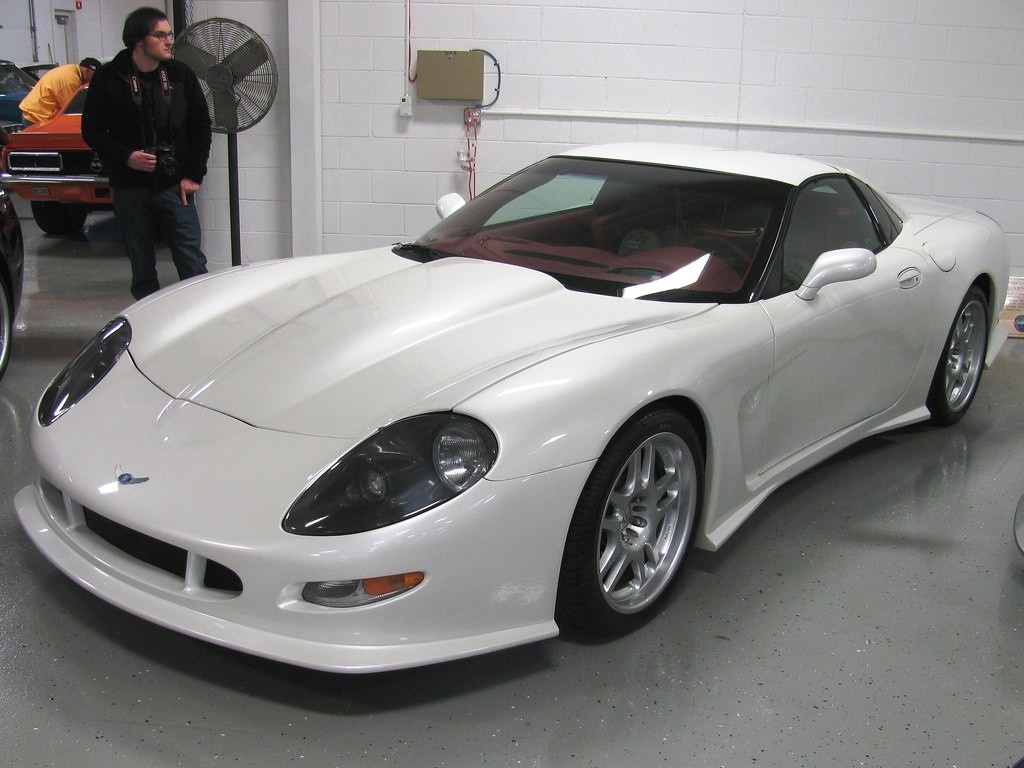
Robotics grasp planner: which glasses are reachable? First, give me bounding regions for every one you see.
[147,31,173,41]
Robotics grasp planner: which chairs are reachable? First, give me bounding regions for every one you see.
[578,189,682,252]
[6,78,24,89]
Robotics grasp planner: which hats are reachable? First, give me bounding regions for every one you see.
[79,58,102,72]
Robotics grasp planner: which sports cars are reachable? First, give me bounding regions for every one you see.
[13,142,1012,679]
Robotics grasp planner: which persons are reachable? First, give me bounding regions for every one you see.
[82,7,212,301]
[18,57,103,130]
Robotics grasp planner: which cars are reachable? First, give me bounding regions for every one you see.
[0,76,121,236]
[0,60,44,126]
[0,184,24,382]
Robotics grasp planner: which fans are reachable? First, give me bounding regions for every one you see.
[170,18,278,267]
